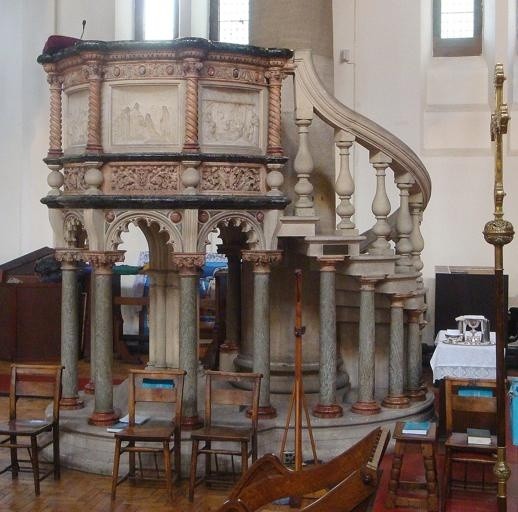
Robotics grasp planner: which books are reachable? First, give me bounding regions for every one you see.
[119,412,152,427]
[401,420,431,436]
[465,426,492,445]
[106,422,129,433]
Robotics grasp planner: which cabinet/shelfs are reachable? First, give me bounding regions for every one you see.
[435,272,508,334]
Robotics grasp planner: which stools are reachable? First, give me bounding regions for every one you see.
[383,420,442,512]
[440,433,499,512]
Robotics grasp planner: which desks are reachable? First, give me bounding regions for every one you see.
[429,330,497,437]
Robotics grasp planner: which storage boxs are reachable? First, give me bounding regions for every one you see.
[505,375,518,446]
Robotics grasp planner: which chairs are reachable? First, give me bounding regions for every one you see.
[0,363,66,496]
[188,370,263,503]
[109,368,186,502]
[212,425,392,512]
